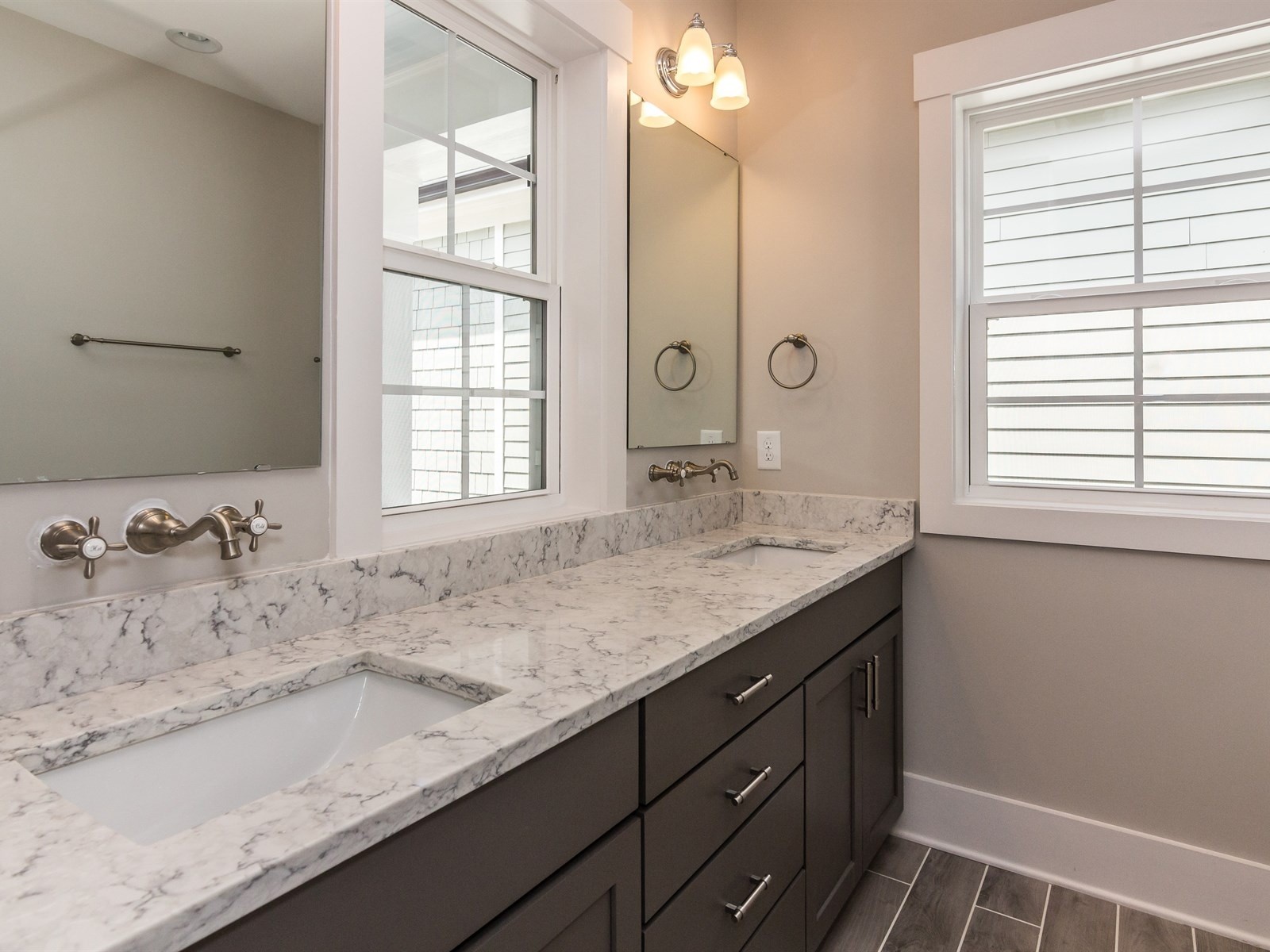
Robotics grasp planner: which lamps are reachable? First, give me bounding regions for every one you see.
[656,12,750,111]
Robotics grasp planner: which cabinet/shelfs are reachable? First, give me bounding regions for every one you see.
[642,557,906,952]
[179,701,634,952]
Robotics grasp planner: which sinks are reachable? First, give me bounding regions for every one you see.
[685,534,859,568]
[10,650,516,851]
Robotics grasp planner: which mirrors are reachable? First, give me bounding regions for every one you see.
[2,2,316,485]
[629,85,739,447]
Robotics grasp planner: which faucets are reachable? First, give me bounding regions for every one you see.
[121,498,244,564]
[666,460,739,483]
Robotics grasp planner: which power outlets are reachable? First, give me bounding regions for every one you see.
[756,430,781,471]
[700,429,723,444]
